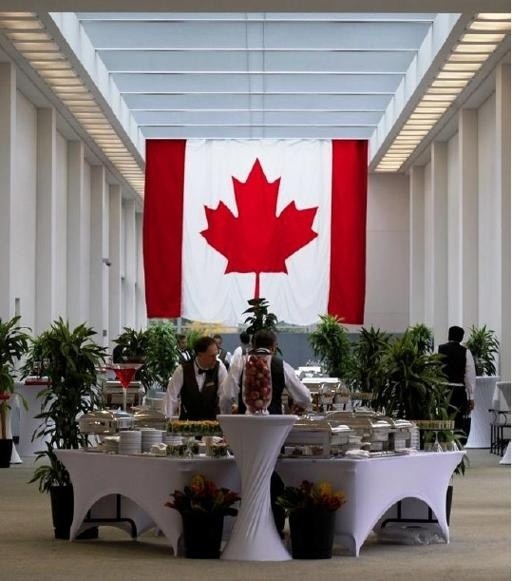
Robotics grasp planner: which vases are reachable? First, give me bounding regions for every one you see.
[182,516,224,559]
[288,517,335,559]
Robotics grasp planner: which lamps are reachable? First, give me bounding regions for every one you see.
[366,12,510,175]
[0,11,144,208]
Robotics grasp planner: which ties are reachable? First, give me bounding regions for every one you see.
[184,350,189,360]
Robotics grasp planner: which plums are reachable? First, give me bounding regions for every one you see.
[243,355,272,408]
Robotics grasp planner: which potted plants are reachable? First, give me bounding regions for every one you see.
[16,315,112,539]
[0,315,32,468]
[368,329,470,527]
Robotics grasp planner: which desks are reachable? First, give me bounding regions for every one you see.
[217,414,298,562]
[496,382,511,465]
[11,381,97,456]
[464,376,500,448]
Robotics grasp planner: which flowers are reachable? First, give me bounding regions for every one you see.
[274,480,348,517]
[164,476,240,516]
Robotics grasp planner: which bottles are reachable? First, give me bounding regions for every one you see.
[241,347,273,416]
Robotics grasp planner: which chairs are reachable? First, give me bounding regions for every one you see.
[489,409,511,457]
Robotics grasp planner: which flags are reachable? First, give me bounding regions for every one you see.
[141,136,368,327]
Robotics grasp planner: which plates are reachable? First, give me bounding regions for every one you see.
[118,428,185,456]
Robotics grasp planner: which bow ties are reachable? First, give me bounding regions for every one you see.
[198,368,208,375]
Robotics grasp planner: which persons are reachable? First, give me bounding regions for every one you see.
[218,332,313,417]
[438,325,478,449]
[167,336,233,421]
[168,332,284,371]
